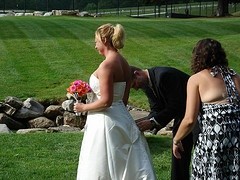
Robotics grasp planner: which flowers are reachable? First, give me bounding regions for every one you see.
[66,80,94,115]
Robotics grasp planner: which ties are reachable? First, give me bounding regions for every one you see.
[148,86,157,98]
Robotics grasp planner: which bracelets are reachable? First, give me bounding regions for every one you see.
[173,136,182,146]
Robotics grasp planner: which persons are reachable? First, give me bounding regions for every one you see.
[173,37,240,180]
[74,22,157,180]
[128,65,198,180]
